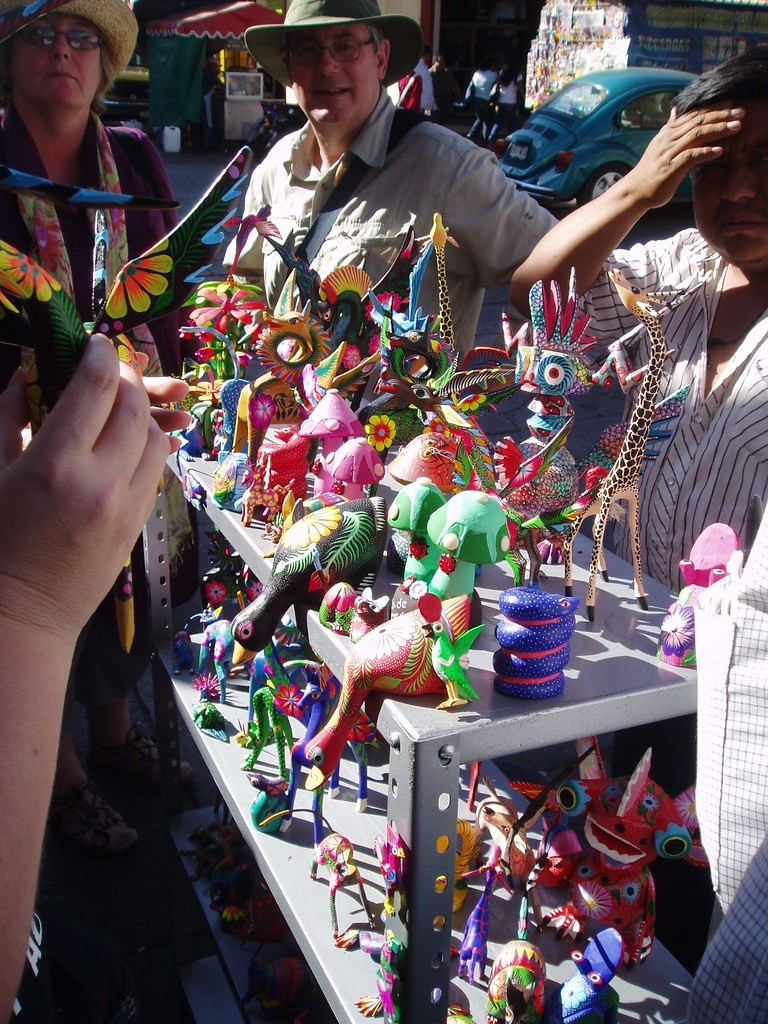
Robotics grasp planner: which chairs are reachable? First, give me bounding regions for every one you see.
[620,98,645,128]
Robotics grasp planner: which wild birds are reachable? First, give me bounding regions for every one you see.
[421,621,487,710]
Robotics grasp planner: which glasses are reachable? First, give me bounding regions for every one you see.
[18,25,105,51]
[290,38,375,62]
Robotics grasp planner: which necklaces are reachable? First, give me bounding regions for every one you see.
[702,300,765,366]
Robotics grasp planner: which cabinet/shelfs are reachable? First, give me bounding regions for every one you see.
[129,387,707,1024]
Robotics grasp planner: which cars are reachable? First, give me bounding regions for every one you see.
[493,64,700,234]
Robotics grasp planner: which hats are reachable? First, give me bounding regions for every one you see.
[0,0,138,98]
[244,0,424,88]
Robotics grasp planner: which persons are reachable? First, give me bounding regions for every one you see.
[1,329,186,1024]
[0,0,201,382]
[205,0,557,369]
[505,50,767,592]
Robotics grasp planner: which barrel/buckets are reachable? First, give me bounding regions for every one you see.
[163,125,181,154]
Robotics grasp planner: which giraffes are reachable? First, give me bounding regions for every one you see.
[415,212,460,344]
[562,268,687,620]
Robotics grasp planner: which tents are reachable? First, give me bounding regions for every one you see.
[148,0,290,41]
